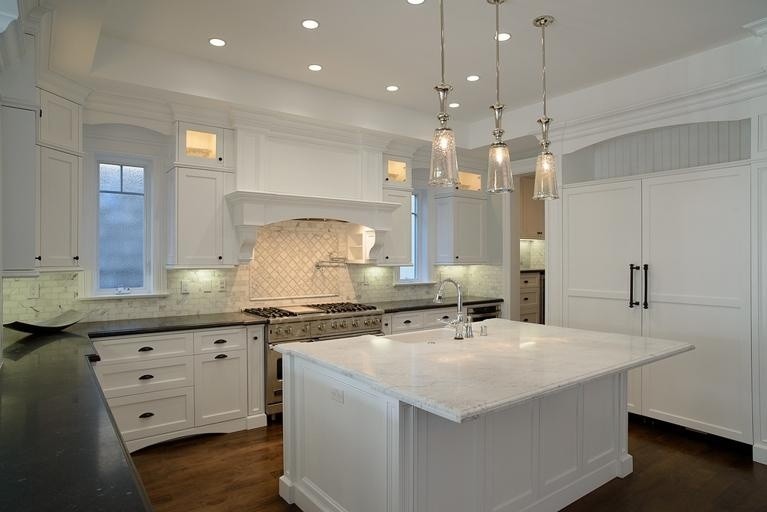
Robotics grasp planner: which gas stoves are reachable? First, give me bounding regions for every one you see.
[242,301,384,342]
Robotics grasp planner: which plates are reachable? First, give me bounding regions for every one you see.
[2,307,87,333]
[3,330,84,363]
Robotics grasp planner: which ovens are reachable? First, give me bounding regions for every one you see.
[264,330,383,416]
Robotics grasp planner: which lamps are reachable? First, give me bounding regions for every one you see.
[533,16,557,200]
[428,1,460,185]
[486,0,515,193]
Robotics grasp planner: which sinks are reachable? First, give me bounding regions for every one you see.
[378,326,458,346]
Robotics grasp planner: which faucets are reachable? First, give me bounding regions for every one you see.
[431,276,464,339]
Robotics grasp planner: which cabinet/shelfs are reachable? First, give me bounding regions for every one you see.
[193,326,264,426]
[90,332,194,441]
[163,168,223,265]
[561,167,752,444]
[433,170,502,265]
[382,306,466,334]
[346,153,412,268]
[39,88,79,154]
[164,123,223,170]
[520,179,544,240]
[36,146,80,271]
[0,20,42,278]
[521,271,540,322]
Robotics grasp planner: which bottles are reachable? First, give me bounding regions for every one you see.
[480,320,487,336]
[465,316,472,338]
